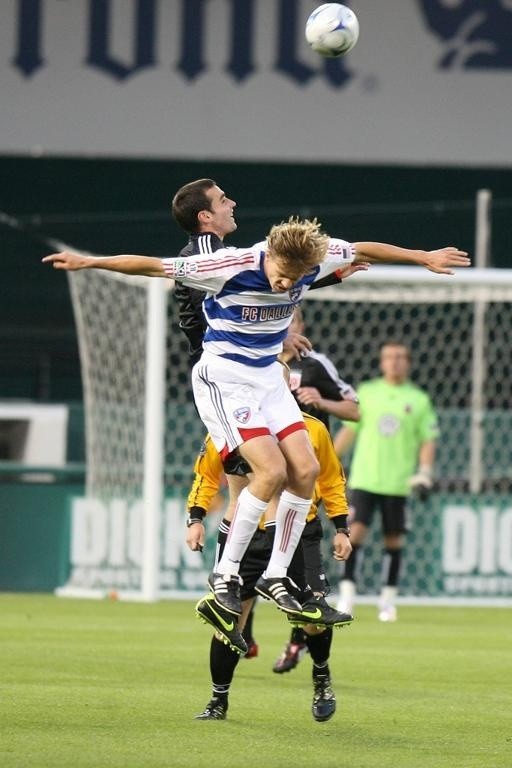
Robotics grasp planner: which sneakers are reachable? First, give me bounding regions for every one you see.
[336,580,360,618]
[271,625,310,676]
[194,692,231,721]
[192,591,253,656]
[242,636,258,662]
[206,569,244,618]
[377,585,399,623]
[251,568,303,616]
[284,591,357,632]
[309,663,338,725]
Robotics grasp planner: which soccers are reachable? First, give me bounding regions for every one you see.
[305,2,359,57]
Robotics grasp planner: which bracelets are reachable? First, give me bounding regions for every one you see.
[335,527,351,537]
[185,518,203,527]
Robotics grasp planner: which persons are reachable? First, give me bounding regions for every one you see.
[171,176,354,657]
[328,341,440,624]
[38,214,471,656]
[187,360,352,723]
[241,304,360,676]
